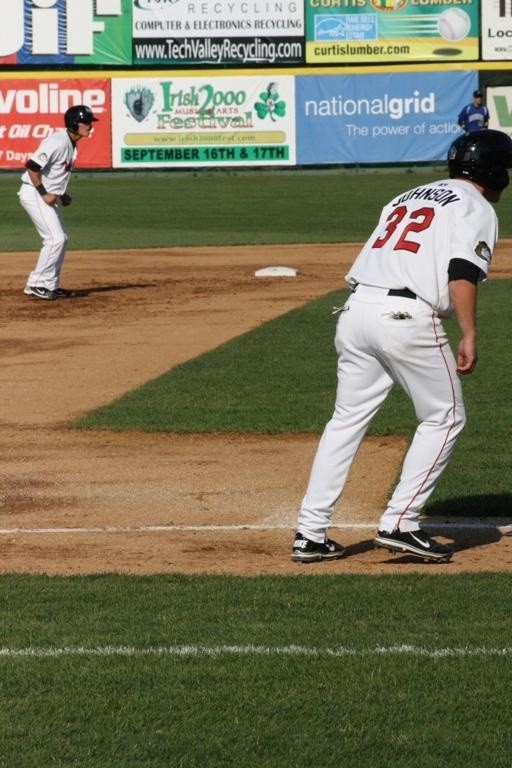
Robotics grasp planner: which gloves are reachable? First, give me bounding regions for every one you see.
[59,192,73,209]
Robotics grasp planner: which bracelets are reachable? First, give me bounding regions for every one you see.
[36,184,48,195]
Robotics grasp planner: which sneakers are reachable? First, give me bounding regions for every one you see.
[21,283,74,301]
[373,527,455,563]
[288,531,348,562]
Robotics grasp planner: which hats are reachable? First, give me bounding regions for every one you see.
[472,90,484,98]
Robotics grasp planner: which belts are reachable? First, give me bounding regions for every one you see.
[350,281,419,301]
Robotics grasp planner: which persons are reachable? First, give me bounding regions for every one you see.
[286,128,511,564]
[14,103,101,300]
[456,90,492,132]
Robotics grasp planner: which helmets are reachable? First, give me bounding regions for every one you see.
[446,129,512,192]
[62,104,100,135]
